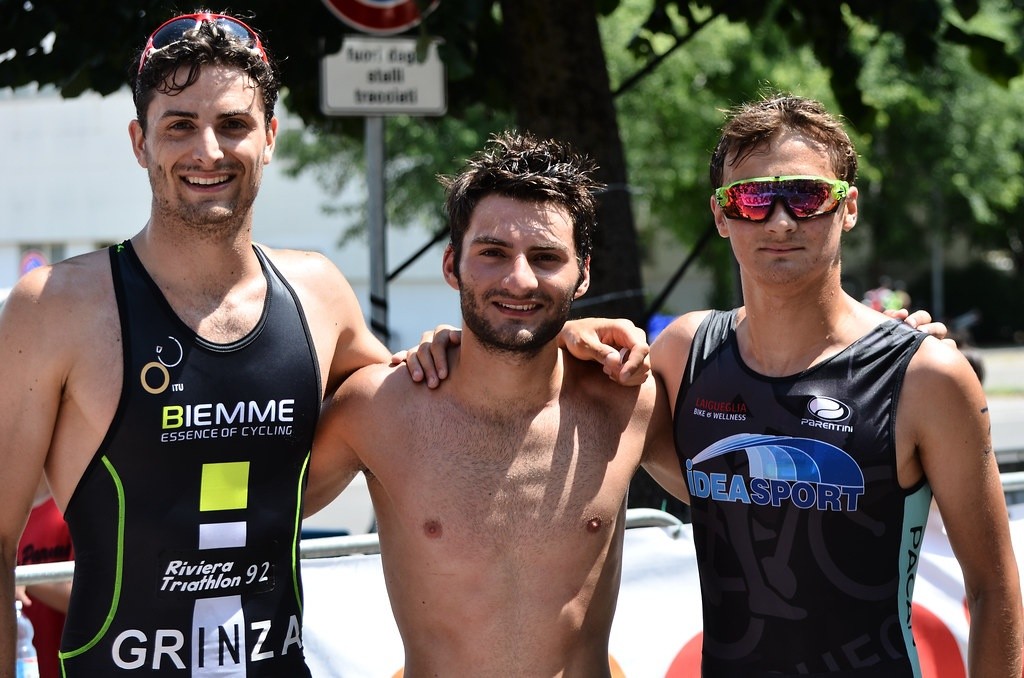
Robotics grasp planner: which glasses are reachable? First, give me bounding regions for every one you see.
[136,12,274,78]
[712,174,851,222]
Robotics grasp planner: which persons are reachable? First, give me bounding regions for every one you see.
[302,132,956,678]
[14,475,79,678]
[392,92,1023,677]
[0,10,651,678]
